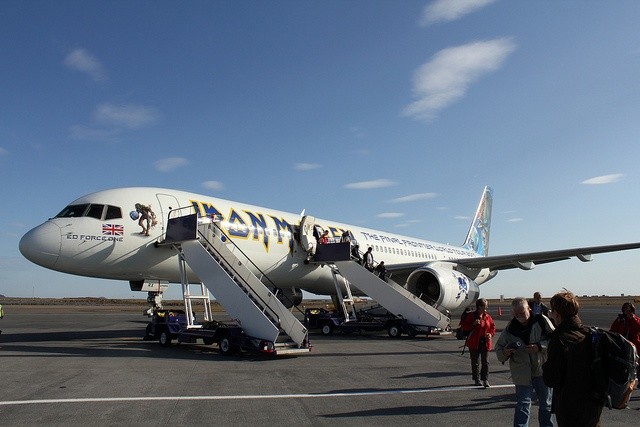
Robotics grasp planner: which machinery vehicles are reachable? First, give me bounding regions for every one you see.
[305,308,406,337]
[143,309,244,354]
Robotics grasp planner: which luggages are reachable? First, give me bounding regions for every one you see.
[456,327,466,340]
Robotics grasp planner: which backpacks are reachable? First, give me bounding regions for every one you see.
[579,323,639,411]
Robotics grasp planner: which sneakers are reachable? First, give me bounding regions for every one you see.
[483,380,491,387]
[475,379,482,385]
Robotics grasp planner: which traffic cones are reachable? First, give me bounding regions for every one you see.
[498,306,503,318]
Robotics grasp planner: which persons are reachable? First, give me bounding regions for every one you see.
[374,261,387,281]
[353,244,361,265]
[462,298,496,388]
[319,230,330,243]
[610,302,639,387]
[340,229,351,242]
[497,297,555,426]
[528,292,548,315]
[363,247,374,273]
[459,305,473,326]
[133,203,157,236]
[542,292,614,426]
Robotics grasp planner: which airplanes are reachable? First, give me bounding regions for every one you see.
[18,185,640,355]
